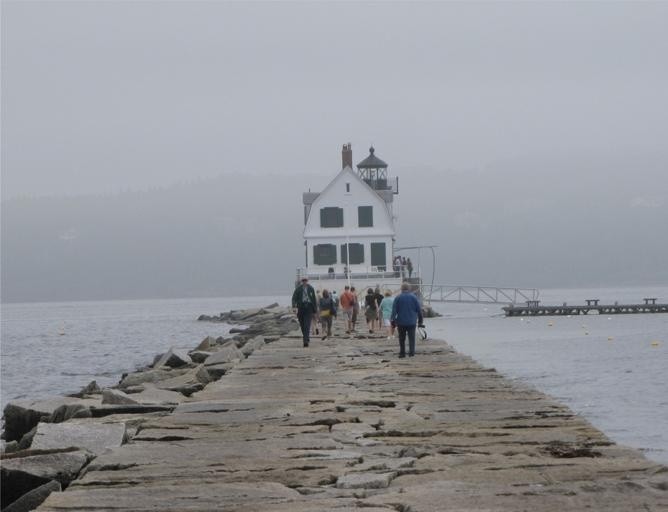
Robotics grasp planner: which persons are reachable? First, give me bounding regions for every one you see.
[292,276,317,347]
[391,283,424,358]
[393,255,413,278]
[310,285,395,340]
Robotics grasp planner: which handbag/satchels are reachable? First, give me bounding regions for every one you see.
[320,309,332,319]
